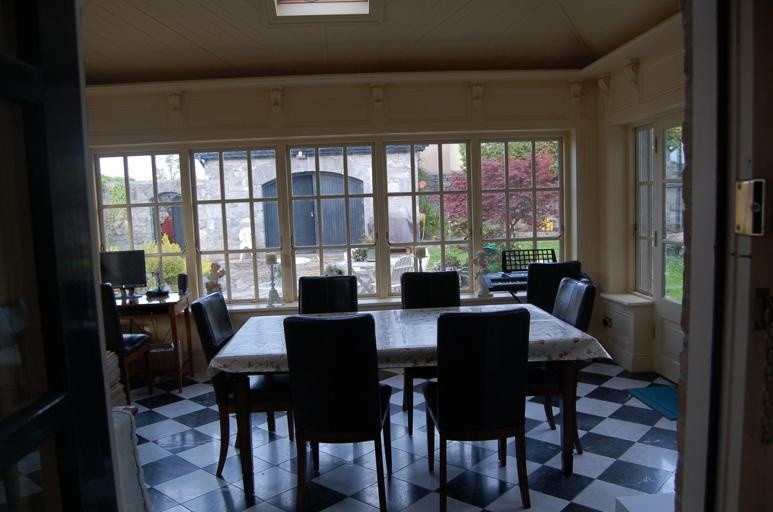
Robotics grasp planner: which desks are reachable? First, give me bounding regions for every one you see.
[112,292,195,394]
[210,303,595,506]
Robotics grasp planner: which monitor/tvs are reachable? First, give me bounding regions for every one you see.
[100,250,146,299]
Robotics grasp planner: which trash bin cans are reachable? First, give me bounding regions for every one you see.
[150,344,174,376]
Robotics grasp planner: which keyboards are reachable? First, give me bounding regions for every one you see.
[115,308,159,316]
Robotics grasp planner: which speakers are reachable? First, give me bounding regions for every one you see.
[177,274,187,295]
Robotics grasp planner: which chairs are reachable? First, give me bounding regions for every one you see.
[495,260,596,466]
[100,283,153,407]
[191,291,291,480]
